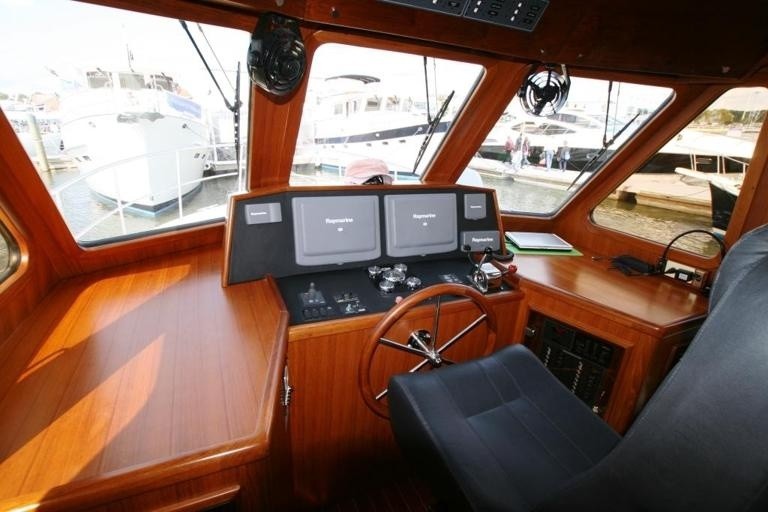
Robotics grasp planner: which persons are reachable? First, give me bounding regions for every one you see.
[504,132,570,172]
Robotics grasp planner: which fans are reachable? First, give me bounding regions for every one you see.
[516,61,573,118]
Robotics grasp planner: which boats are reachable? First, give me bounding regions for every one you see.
[0,44,756,218]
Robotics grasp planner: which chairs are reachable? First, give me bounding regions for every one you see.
[388,224,768,512]
